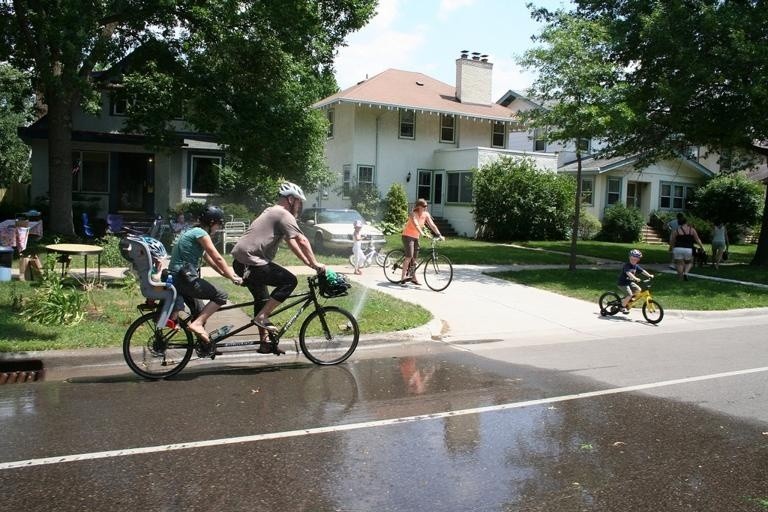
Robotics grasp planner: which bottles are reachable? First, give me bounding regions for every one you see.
[210,324,236,342]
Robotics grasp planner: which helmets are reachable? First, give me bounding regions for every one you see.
[354,222,360,228]
[201,206,226,227]
[628,249,643,259]
[140,236,167,263]
[278,181,306,201]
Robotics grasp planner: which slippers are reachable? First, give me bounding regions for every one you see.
[412,281,423,285]
[256,349,285,354]
[215,348,224,355]
[186,324,210,345]
[401,278,412,285]
[251,319,278,331]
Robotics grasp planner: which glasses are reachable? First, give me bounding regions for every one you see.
[421,204,428,207]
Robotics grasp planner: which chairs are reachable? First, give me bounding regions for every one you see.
[235,217,250,230]
[222,213,234,228]
[222,221,245,252]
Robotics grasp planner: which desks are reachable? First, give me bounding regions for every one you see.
[44,242,103,286]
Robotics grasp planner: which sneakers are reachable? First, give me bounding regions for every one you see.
[170,316,181,329]
[620,308,629,313]
[683,273,689,280]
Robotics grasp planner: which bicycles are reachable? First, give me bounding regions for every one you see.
[597,276,664,325]
[348,236,390,269]
[383,234,454,293]
[121,268,361,379]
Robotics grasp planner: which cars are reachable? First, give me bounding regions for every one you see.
[296,208,387,253]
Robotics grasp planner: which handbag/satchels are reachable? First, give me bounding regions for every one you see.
[319,272,351,298]
[693,248,707,267]
[722,251,728,261]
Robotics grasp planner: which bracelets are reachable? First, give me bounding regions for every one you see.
[436,232,441,237]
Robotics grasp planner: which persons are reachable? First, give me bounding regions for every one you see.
[167,204,247,355]
[231,180,326,354]
[402,199,445,285]
[171,214,191,233]
[670,217,703,280]
[711,219,729,271]
[618,249,654,314]
[667,213,684,270]
[352,219,366,274]
[138,235,185,330]
